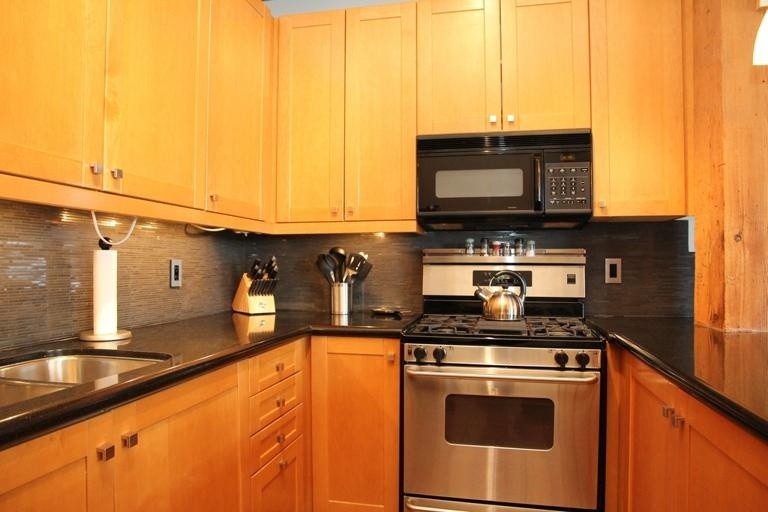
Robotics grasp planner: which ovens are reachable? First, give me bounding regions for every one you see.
[401,364,601,512]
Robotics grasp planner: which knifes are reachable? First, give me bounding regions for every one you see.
[245,252,280,296]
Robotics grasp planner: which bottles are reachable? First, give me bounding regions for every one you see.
[465,237,536,257]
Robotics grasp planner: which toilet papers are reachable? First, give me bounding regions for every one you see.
[93,249,118,339]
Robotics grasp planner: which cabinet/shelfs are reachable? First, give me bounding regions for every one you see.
[615,349,767,511]
[277,0,417,231]
[243,339,311,512]
[314,334,401,512]
[416,0,591,138]
[2,0,202,211]
[203,0,277,220]
[1,361,244,512]
[590,0,688,224]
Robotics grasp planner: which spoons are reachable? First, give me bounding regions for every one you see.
[328,247,345,283]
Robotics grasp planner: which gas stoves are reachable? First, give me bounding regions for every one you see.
[402,313,607,371]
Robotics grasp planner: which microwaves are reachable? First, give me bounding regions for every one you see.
[415,128,593,233]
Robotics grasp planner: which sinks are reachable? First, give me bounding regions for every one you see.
[1,354,163,386]
[0,377,71,409]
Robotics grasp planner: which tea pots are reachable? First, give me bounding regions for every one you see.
[473,271,527,322]
